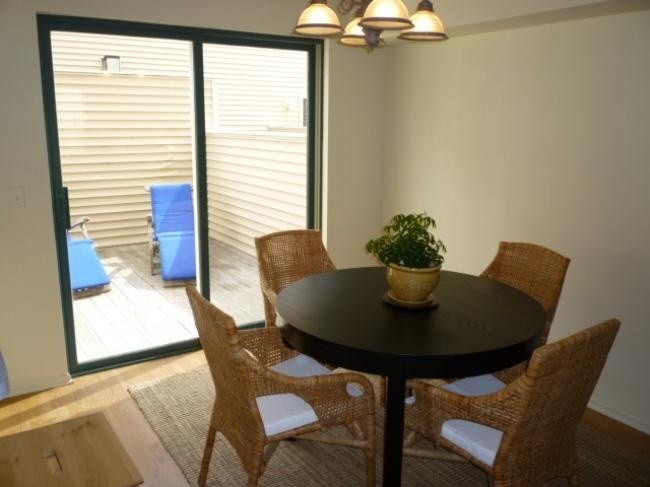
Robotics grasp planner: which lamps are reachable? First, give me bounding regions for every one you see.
[293,0,448,52]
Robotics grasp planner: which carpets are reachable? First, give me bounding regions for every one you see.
[122,337,648,485]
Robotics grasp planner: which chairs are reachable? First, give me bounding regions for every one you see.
[144,181,198,283]
[401,314,620,486]
[182,278,378,487]
[65,215,112,295]
[252,226,339,370]
[476,238,573,383]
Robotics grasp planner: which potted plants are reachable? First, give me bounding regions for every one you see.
[367,211,445,311]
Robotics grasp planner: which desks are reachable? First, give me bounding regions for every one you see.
[272,263,546,481]
